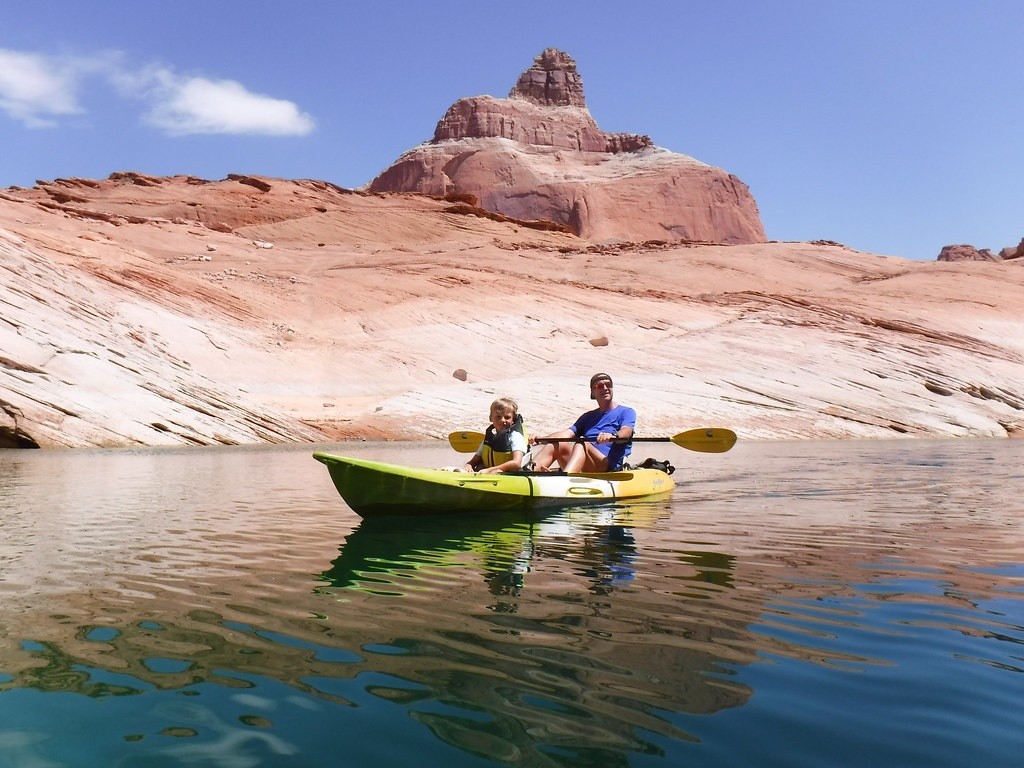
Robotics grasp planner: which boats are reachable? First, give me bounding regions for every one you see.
[312,451,679,521]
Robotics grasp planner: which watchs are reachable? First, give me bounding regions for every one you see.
[611,432,619,440]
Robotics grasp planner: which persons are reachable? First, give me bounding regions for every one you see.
[530,373,636,473]
[464,398,532,476]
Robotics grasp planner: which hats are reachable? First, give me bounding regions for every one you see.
[589,373,612,400]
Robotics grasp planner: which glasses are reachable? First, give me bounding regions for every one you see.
[594,381,613,391]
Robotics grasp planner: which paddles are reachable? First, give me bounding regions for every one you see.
[449,427,737,453]
